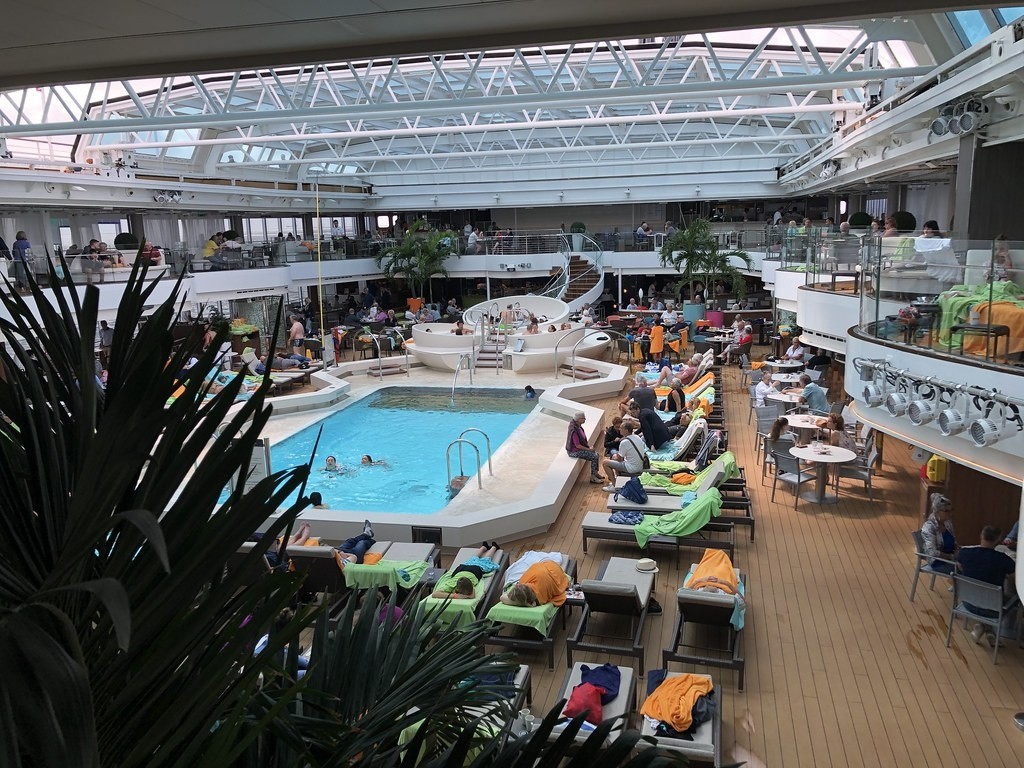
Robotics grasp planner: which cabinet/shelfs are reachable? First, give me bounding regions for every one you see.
[919,477,945,530]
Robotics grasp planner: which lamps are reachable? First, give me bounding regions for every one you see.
[494,193,500,205]
[820,161,838,179]
[624,189,630,198]
[66,184,86,199]
[695,185,700,197]
[862,370,1024,449]
[557,192,564,203]
[430,196,439,202]
[228,195,336,207]
[928,96,991,143]
[152,190,183,205]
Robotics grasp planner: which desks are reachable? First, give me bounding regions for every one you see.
[779,415,828,465]
[569,312,782,364]
[358,334,391,361]
[909,300,941,348]
[477,238,497,255]
[832,270,859,294]
[334,325,355,349]
[766,393,801,438]
[770,372,802,413]
[384,326,408,356]
[712,233,729,251]
[647,233,668,252]
[960,544,1024,640]
[763,360,804,391]
[789,444,857,504]
[361,321,378,326]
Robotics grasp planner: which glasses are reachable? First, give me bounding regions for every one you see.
[579,418,585,420]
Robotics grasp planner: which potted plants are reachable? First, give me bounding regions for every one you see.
[571,222,586,252]
[374,219,461,313]
[657,214,754,341]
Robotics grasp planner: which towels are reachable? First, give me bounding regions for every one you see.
[483,550,573,638]
[161,359,279,406]
[778,324,791,337]
[303,241,315,249]
[751,362,766,371]
[633,325,687,360]
[414,566,497,634]
[676,548,746,632]
[277,535,431,589]
[639,673,713,732]
[635,371,716,427]
[334,326,396,350]
[785,226,821,274]
[934,280,1024,357]
[633,450,740,550]
[861,246,875,262]
[395,312,415,349]
[229,317,260,354]
[888,238,963,283]
[644,438,684,461]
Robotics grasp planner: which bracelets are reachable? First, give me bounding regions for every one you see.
[454,594,458,598]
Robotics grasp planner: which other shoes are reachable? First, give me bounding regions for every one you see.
[646,597,662,615]
[716,355,723,358]
[595,473,605,479]
[590,477,604,484]
[724,363,730,366]
[986,630,1002,647]
[971,624,984,642]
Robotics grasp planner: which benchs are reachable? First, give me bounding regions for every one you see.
[0,236,396,296]
[816,229,1024,357]
[949,322,1010,365]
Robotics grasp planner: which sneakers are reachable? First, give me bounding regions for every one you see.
[608,482,613,485]
[603,486,615,492]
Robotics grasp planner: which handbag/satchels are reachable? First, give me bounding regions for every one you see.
[643,456,650,469]
[562,681,608,725]
[613,476,648,504]
[659,357,672,372]
[666,468,694,478]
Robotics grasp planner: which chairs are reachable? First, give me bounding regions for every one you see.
[604,234,616,250]
[787,236,808,267]
[243,246,268,269]
[740,353,880,554]
[633,232,649,251]
[910,530,1021,665]
[286,306,461,359]
[320,242,335,260]
[831,240,861,294]
[218,247,243,271]
[460,235,517,256]
[165,347,755,768]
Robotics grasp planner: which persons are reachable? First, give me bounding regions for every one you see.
[922,493,1019,647]
[525,385,536,399]
[432,541,500,598]
[334,520,376,566]
[247,521,311,554]
[310,492,323,509]
[228,154,234,163]
[326,456,337,469]
[202,232,243,270]
[636,206,1014,282]
[484,281,858,483]
[690,548,736,595]
[0,231,33,292]
[279,154,288,168]
[362,455,372,464]
[499,561,570,606]
[272,220,514,259]
[0,286,475,444]
[64,239,161,285]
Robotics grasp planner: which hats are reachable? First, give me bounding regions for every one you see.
[635,558,659,573]
